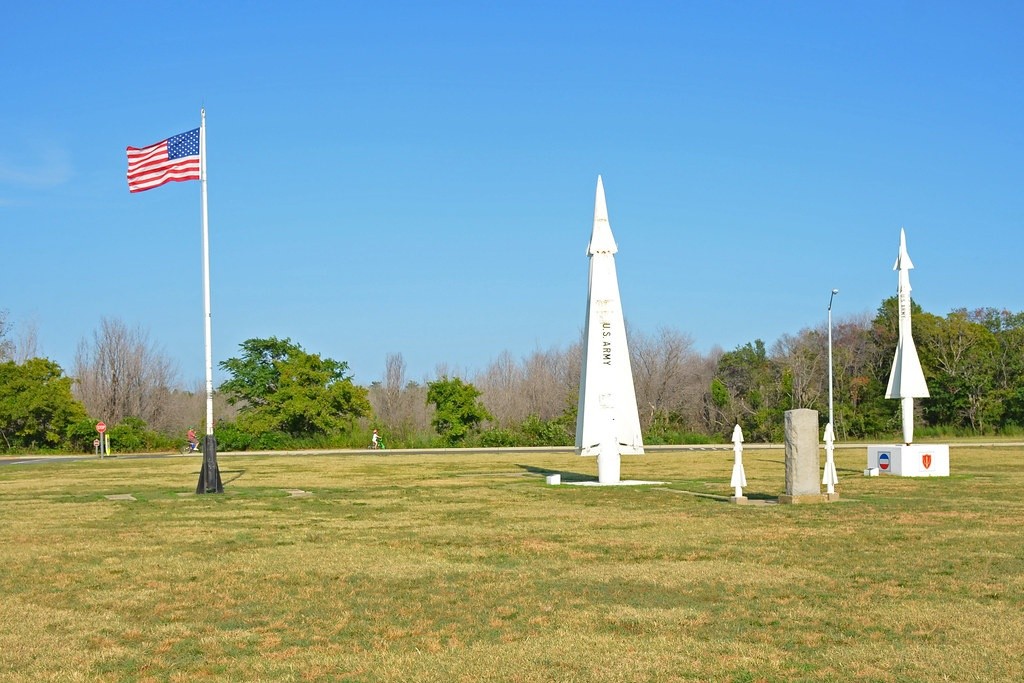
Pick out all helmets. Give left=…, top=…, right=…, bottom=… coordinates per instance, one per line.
left=188, top=425, right=193, bottom=430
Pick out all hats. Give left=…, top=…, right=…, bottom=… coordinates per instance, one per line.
left=373, top=429, right=377, bottom=434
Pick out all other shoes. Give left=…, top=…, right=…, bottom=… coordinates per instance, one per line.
left=193, top=448, right=199, bottom=450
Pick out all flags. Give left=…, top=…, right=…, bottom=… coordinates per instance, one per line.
left=127, top=127, right=204, bottom=194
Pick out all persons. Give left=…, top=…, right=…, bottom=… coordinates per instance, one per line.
left=188, top=426, right=200, bottom=450
left=372, top=430, right=382, bottom=448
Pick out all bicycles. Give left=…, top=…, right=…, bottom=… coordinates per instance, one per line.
left=179, top=441, right=205, bottom=454
left=367, top=437, right=386, bottom=449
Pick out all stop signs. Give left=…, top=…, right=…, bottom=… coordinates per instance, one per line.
left=96, top=422, right=107, bottom=433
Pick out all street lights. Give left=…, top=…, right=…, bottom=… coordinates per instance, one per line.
left=828, top=289, right=838, bottom=443
